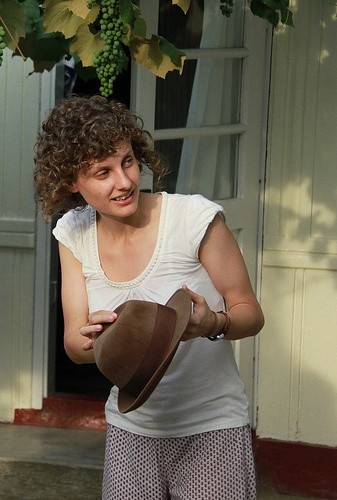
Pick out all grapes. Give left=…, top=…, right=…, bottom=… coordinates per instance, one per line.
left=87, top=0, right=129, bottom=98
left=0, top=26, right=6, bottom=67
left=219, top=0, right=234, bottom=18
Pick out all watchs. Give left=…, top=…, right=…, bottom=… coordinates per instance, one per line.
left=206, top=310, right=231, bottom=341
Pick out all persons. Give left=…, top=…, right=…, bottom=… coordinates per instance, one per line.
left=33, top=96, right=264, bottom=500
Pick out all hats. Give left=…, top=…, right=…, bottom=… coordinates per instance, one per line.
left=90, top=289, right=195, bottom=414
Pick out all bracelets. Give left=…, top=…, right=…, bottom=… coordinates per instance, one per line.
left=201, top=309, right=217, bottom=338
